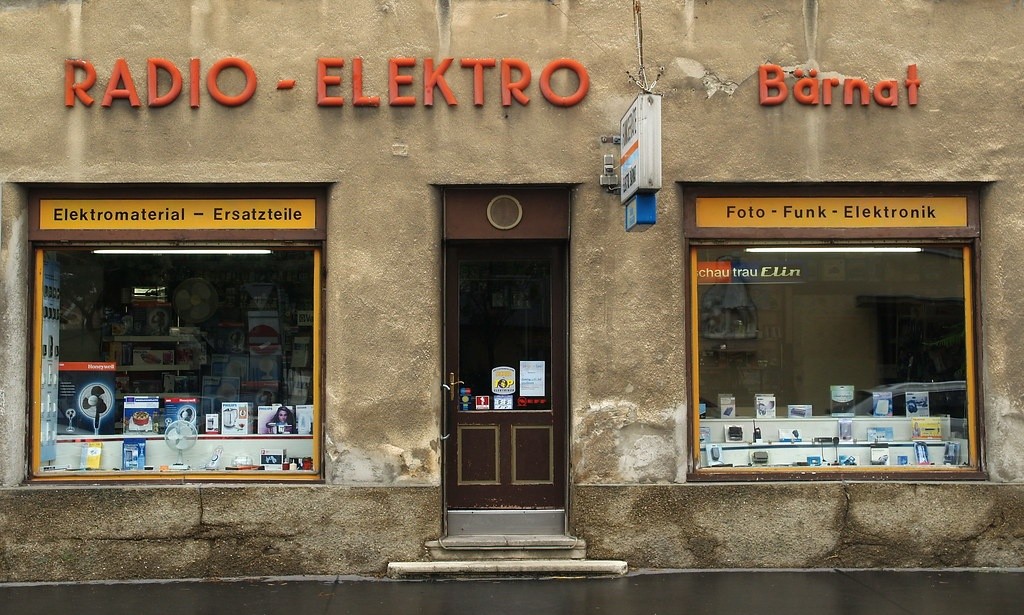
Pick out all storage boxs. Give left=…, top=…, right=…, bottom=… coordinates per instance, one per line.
left=201, top=396, right=221, bottom=418
left=754, top=394, right=775, bottom=418
left=133, top=350, right=174, bottom=365
left=870, top=444, right=890, bottom=466
left=265, top=463, right=289, bottom=471
left=164, top=375, right=198, bottom=393
left=139, top=380, right=163, bottom=393
left=247, top=310, right=279, bottom=344
left=249, top=343, right=282, bottom=356
left=123, top=395, right=159, bottom=434
left=202, top=377, right=240, bottom=402
left=829, top=385, right=855, bottom=417
left=112, top=307, right=171, bottom=335
left=164, top=397, right=200, bottom=428
left=176, top=342, right=207, bottom=365
left=296, top=405, right=314, bottom=435
left=718, top=393, right=735, bottom=418
left=221, top=402, right=253, bottom=434
left=807, top=456, right=821, bottom=467
left=241, top=393, right=276, bottom=413
left=56, top=362, right=116, bottom=435
left=258, top=406, right=292, bottom=435
left=116, top=376, right=129, bottom=394
left=260, top=448, right=286, bottom=466
left=787, top=404, right=812, bottom=418
left=206, top=413, right=220, bottom=434
left=778, top=429, right=802, bottom=442
left=211, top=354, right=250, bottom=381
left=839, top=456, right=858, bottom=467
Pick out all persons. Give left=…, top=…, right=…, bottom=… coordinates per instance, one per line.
left=267, top=407, right=294, bottom=434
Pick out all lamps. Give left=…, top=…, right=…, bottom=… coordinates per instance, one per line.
left=743, top=245, right=924, bottom=254
left=91, top=248, right=273, bottom=254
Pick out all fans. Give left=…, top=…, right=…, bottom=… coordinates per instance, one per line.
left=151, top=311, right=165, bottom=335
left=229, top=332, right=244, bottom=351
left=217, top=384, right=236, bottom=413
left=79, top=383, right=113, bottom=434
left=259, top=359, right=274, bottom=379
left=165, top=420, right=198, bottom=470
left=65, top=409, right=76, bottom=432
left=169, top=277, right=218, bottom=334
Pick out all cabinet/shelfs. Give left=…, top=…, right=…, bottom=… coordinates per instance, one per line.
left=103, top=335, right=201, bottom=428
